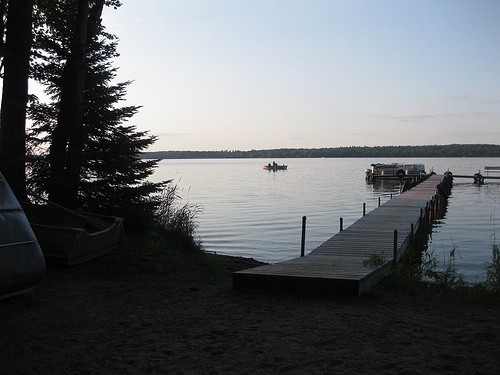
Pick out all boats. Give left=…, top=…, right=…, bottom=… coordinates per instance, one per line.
left=264, top=163, right=288, bottom=170
left=365, top=163, right=427, bottom=181
